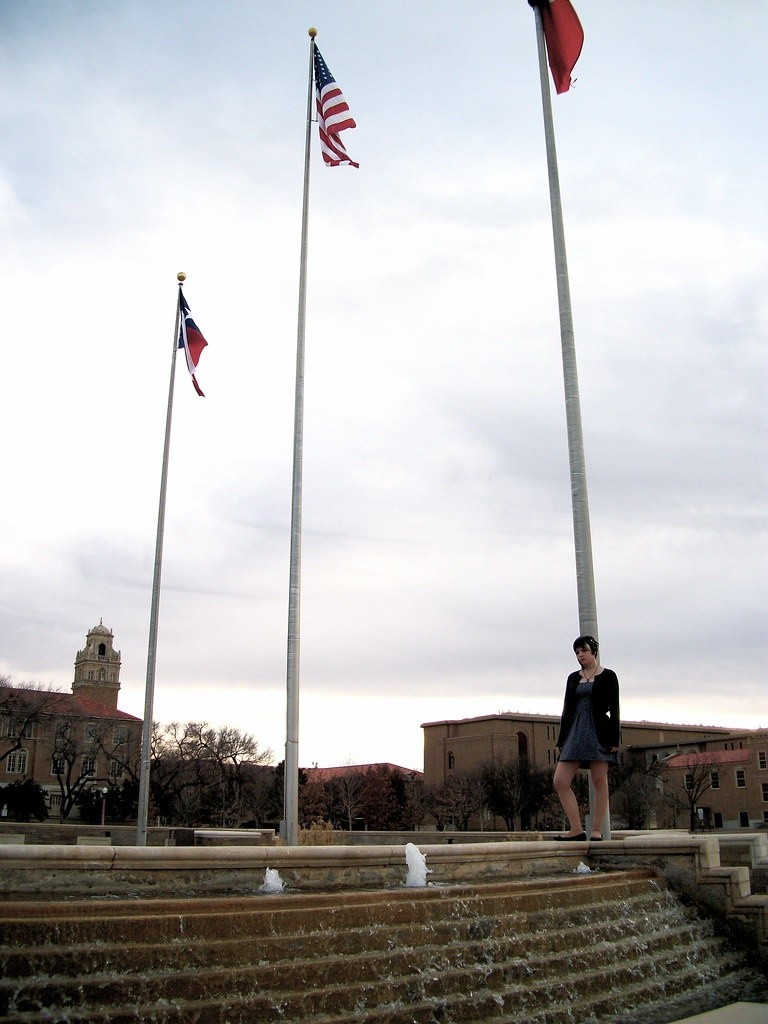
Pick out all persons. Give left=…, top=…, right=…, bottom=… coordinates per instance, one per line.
left=552, top=635, right=621, bottom=841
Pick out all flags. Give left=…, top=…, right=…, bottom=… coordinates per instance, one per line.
left=312, top=40, right=360, bottom=169
left=527, top=0, right=586, bottom=95
left=178, top=282, right=210, bottom=397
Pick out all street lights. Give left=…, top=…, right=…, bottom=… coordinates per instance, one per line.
left=101, top=786, right=107, bottom=824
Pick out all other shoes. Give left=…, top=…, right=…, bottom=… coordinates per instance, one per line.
left=552, top=832, right=587, bottom=841
left=590, top=834, right=604, bottom=840
